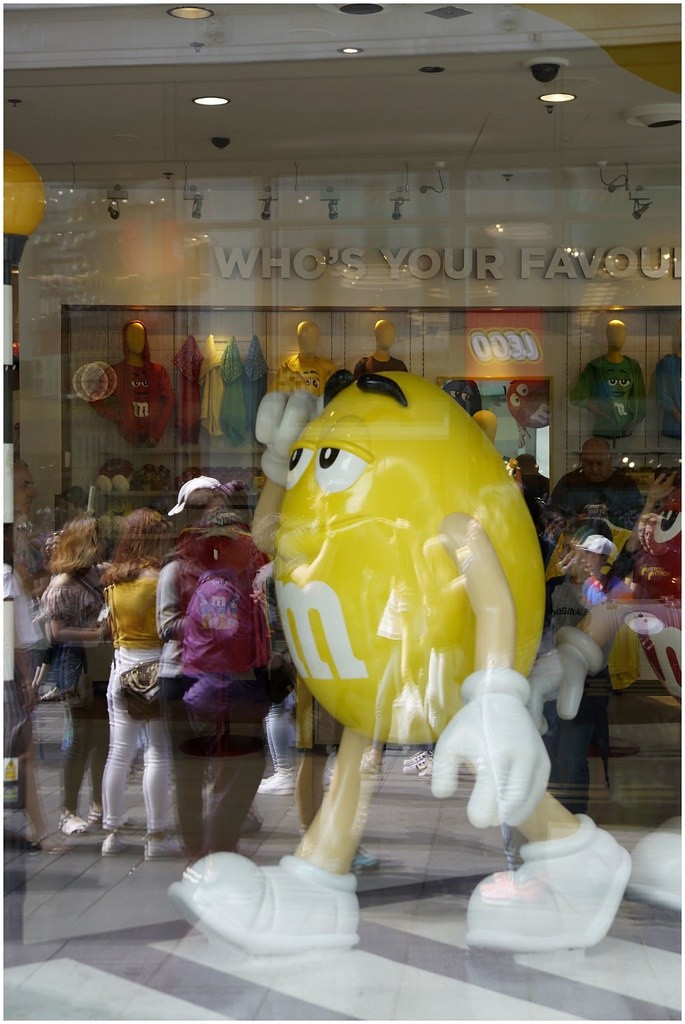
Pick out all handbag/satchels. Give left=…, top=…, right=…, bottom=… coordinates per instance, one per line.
left=66, top=673, right=94, bottom=709
left=119, top=659, right=164, bottom=720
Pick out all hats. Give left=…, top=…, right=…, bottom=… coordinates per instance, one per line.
left=579, top=536, right=618, bottom=557
left=168, top=475, right=221, bottom=516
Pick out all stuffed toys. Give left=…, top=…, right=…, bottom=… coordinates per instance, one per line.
left=179, top=371, right=630, bottom=957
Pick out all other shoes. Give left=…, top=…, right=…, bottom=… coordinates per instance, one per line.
left=41, top=681, right=75, bottom=701
left=202, top=808, right=262, bottom=835
left=58, top=811, right=89, bottom=835
left=145, top=835, right=184, bottom=861
left=88, top=809, right=103, bottom=824
left=101, top=830, right=127, bottom=854
left=349, top=845, right=380, bottom=869
left=256, top=767, right=296, bottom=796
left=30, top=829, right=70, bottom=854
left=358, top=751, right=382, bottom=774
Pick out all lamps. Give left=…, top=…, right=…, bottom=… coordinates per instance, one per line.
left=320, top=191, right=340, bottom=219
left=183, top=185, right=204, bottom=219
left=107, top=191, right=128, bottom=220
left=258, top=192, right=279, bottom=220
left=629, top=192, right=654, bottom=221
left=389, top=164, right=411, bottom=220
left=600, top=163, right=630, bottom=193
left=420, top=168, right=444, bottom=194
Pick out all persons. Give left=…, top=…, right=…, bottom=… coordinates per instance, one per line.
left=551, top=436, right=675, bottom=552
left=352, top=319, right=408, bottom=382
left=502, top=515, right=681, bottom=876
left=88, top=320, right=173, bottom=447
left=8, top=458, right=456, bottom=872
left=266, top=321, right=337, bottom=396
left=653, top=320, right=682, bottom=440
left=513, top=455, right=549, bottom=530
left=570, top=320, right=646, bottom=439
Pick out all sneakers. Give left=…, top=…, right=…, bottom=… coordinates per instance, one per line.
left=403, top=750, right=434, bottom=778
